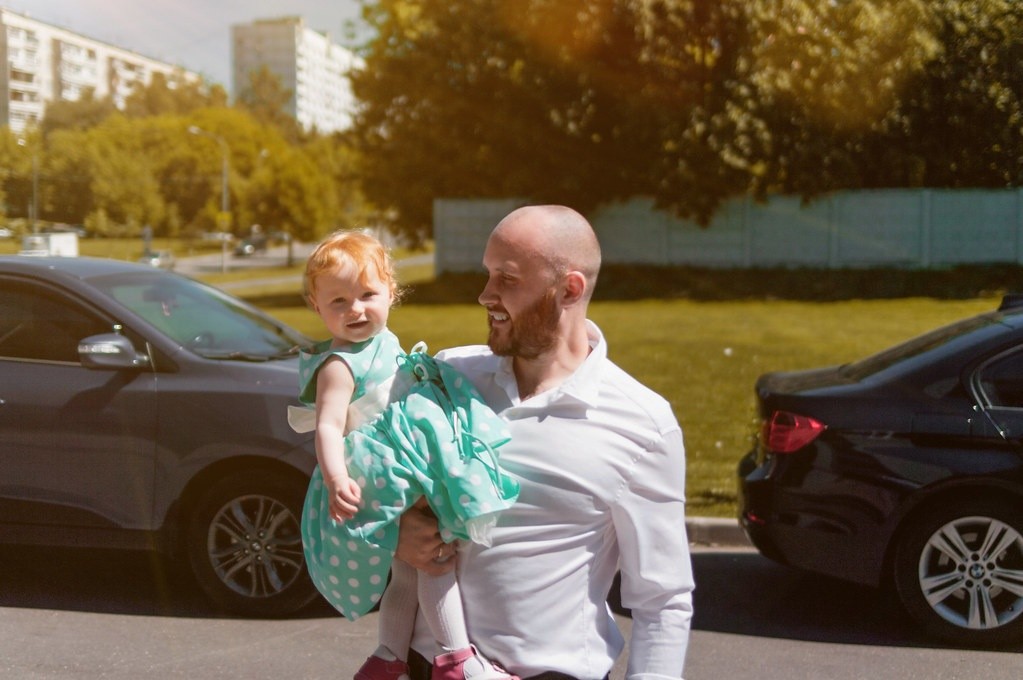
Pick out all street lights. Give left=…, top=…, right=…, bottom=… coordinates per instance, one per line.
left=189, top=125, right=230, bottom=275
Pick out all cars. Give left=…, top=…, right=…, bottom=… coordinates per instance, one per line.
left=736, top=295, right=1023, bottom=650
left=235, top=232, right=289, bottom=255
left=0, top=256, right=339, bottom=618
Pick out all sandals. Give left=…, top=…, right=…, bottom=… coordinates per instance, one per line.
left=353, top=644, right=411, bottom=680
left=431, top=641, right=521, bottom=680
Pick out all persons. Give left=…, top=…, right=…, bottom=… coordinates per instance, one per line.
left=299, top=228, right=521, bottom=680
left=394, top=204, right=697, bottom=679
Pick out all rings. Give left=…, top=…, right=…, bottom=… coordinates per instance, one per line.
left=439, top=548, right=443, bottom=556
